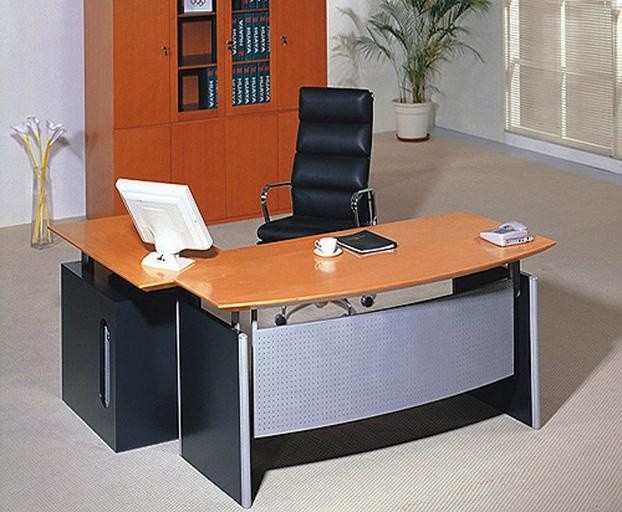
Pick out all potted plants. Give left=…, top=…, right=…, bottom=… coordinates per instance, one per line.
left=337, top=0, right=498, bottom=144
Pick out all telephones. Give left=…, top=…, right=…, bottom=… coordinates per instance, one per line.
left=480, top=221, right=534, bottom=247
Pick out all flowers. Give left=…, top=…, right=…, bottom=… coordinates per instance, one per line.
left=9, top=115, right=68, bottom=250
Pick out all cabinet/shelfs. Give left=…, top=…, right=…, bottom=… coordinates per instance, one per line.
left=79, top=0, right=329, bottom=236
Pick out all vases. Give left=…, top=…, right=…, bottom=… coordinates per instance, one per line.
left=29, top=164, right=55, bottom=250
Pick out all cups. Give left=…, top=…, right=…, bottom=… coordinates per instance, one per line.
left=314, top=237, right=337, bottom=254
left=315, top=262, right=335, bottom=273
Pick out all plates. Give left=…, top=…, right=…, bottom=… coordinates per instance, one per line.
left=314, top=256, right=339, bottom=263
left=312, top=248, right=343, bottom=258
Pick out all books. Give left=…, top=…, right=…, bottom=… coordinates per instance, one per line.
left=336, top=244, right=396, bottom=258
left=198, top=0, right=271, bottom=109
left=334, top=230, right=398, bottom=254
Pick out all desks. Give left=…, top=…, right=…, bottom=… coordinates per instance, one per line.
left=44, top=211, right=557, bottom=511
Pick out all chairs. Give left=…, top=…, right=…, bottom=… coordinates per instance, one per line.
left=250, top=81, right=384, bottom=327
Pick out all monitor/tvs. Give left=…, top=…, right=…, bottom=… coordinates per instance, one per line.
left=113, top=176, right=214, bottom=273
